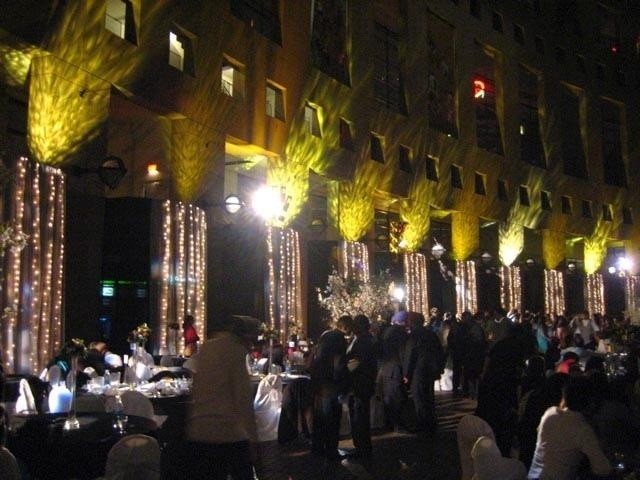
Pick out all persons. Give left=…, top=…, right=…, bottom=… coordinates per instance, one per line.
left=45, top=306, right=639, bottom=480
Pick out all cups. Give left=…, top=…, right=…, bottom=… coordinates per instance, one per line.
left=110, top=372, right=121, bottom=385
left=127, top=377, right=190, bottom=398
left=87, top=376, right=105, bottom=391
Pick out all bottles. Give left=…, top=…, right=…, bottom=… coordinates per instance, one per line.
left=104, top=369, right=110, bottom=385
left=48, top=385, right=72, bottom=412
